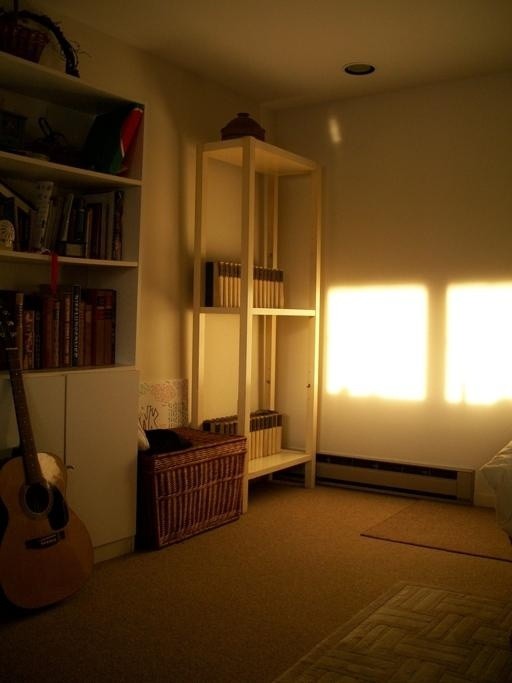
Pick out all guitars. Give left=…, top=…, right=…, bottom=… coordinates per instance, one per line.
left=0, top=305, right=94, bottom=610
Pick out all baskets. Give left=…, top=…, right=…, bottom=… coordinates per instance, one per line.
left=0, top=19, right=51, bottom=64
left=138, top=420, right=245, bottom=549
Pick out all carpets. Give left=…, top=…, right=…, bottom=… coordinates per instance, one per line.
left=360, top=499, right=512, bottom=561
left=270, top=579, right=511, bottom=681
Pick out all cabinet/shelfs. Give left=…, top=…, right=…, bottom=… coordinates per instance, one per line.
left=0, top=48, right=148, bottom=567
left=191, top=135, right=323, bottom=515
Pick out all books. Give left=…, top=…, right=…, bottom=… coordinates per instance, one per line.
left=0, top=97, right=143, bottom=371
